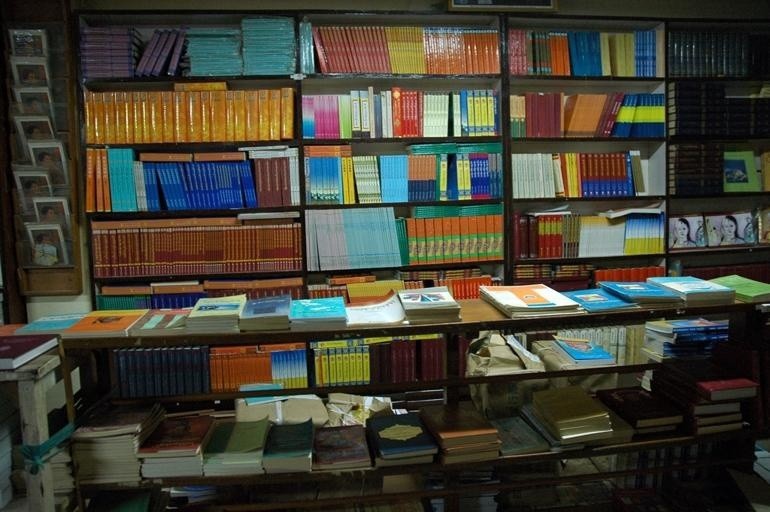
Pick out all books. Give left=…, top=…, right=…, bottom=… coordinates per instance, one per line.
left=80, top=19, right=301, bottom=308
left=507, top=26, right=666, bottom=292
left=303, top=19, right=505, bottom=302
left=2, top=275, right=770, bottom=511
left=666, top=25, right=769, bottom=282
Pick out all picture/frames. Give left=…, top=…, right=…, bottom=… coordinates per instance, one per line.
left=446, top=0, right=560, bottom=14
